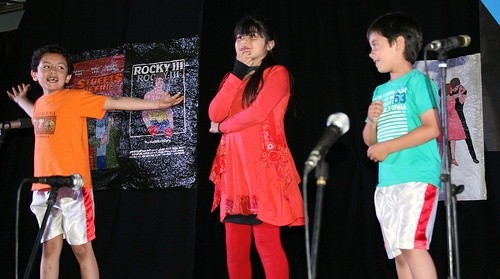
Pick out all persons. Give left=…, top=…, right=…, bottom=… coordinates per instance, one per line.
left=3, top=45, right=184, bottom=279
left=360, top=11, right=443, bottom=279
left=206, top=15, right=310, bottom=279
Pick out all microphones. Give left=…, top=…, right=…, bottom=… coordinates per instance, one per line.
left=424, top=33, right=470, bottom=55
left=302, top=111, right=350, bottom=178
left=0, top=117, right=32, bottom=129
left=27, top=174, right=84, bottom=192
